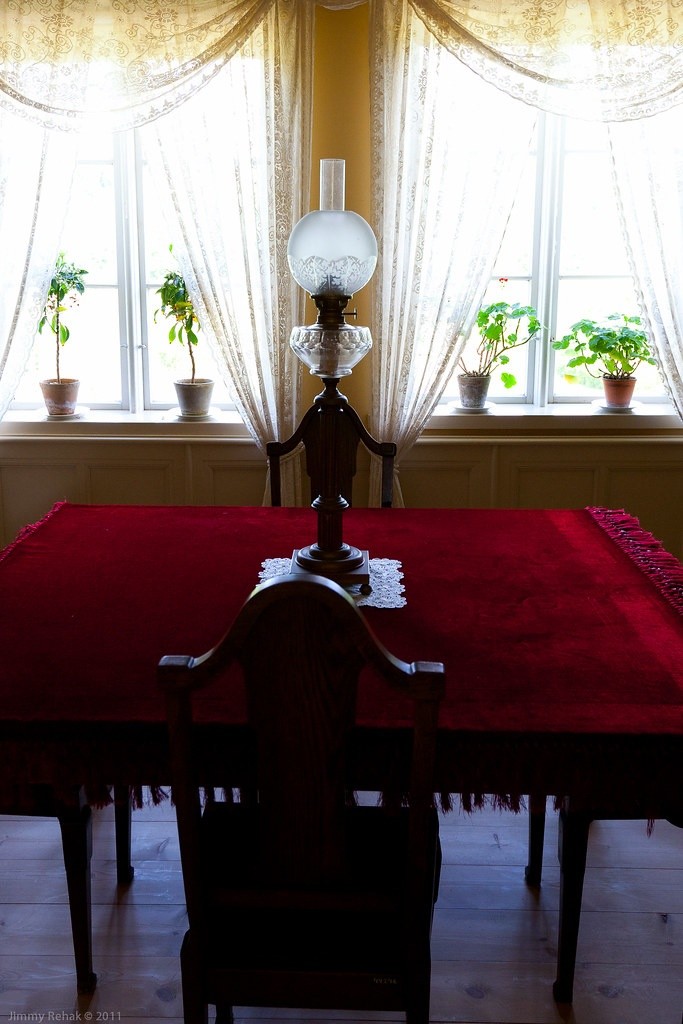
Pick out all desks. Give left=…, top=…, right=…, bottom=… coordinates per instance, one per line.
left=0, top=497, right=683, bottom=1024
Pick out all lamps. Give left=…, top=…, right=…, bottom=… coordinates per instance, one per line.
left=284, top=158, right=382, bottom=597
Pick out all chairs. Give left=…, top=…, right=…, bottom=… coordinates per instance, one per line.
left=263, top=395, right=399, bottom=510
left=152, top=574, right=454, bottom=1024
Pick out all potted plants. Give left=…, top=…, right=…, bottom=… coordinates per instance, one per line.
left=442, top=293, right=550, bottom=413
left=551, top=311, right=659, bottom=412
left=35, top=250, right=92, bottom=420
left=153, top=242, right=222, bottom=422
